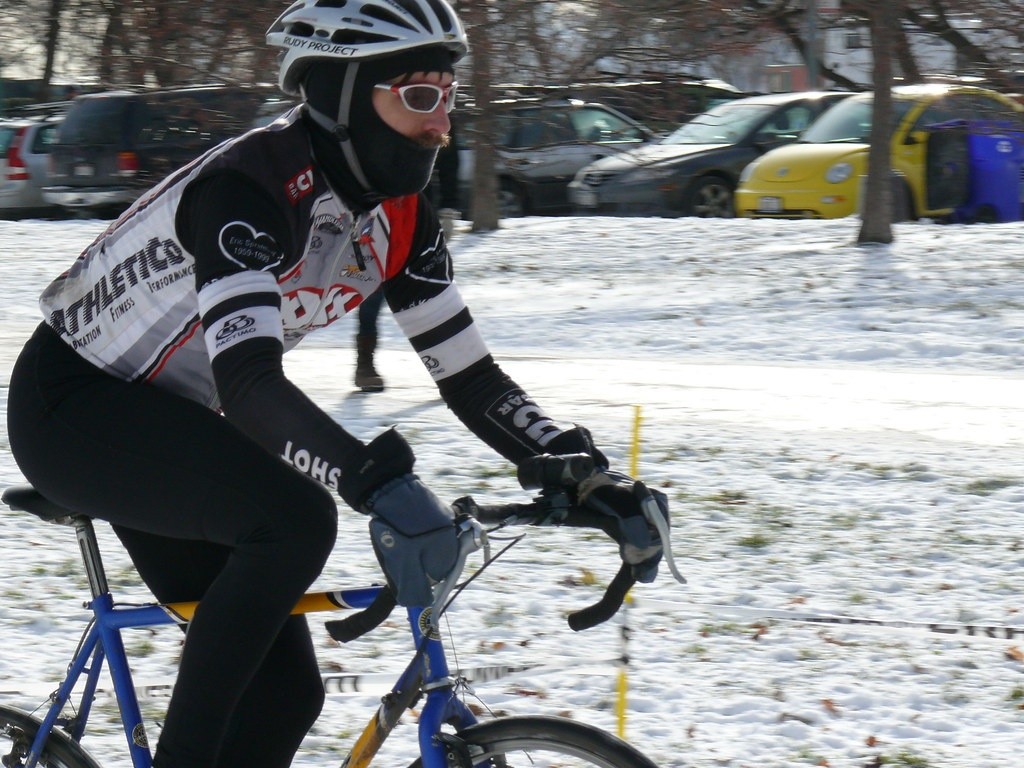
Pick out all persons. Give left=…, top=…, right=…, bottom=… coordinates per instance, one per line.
left=7, top=0, right=669, bottom=768
left=355, top=113, right=458, bottom=391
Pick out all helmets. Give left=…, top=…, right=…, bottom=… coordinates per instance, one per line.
left=264, top=0, right=467, bottom=98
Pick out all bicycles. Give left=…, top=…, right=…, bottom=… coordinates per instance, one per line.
left=0, top=453, right=687, bottom=768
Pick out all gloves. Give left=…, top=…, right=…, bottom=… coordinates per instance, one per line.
left=519, top=444, right=670, bottom=584
left=338, top=426, right=457, bottom=609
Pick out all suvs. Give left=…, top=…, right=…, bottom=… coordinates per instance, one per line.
left=446, top=72, right=754, bottom=219
left=1, top=83, right=300, bottom=219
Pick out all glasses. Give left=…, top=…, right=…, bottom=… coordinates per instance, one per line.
left=377, top=81, right=459, bottom=113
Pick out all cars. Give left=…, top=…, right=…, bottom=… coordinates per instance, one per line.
left=565, top=88, right=957, bottom=218
left=735, top=75, right=1024, bottom=223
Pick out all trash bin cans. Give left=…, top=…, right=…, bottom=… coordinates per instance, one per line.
left=924, top=117, right=1023, bottom=222
